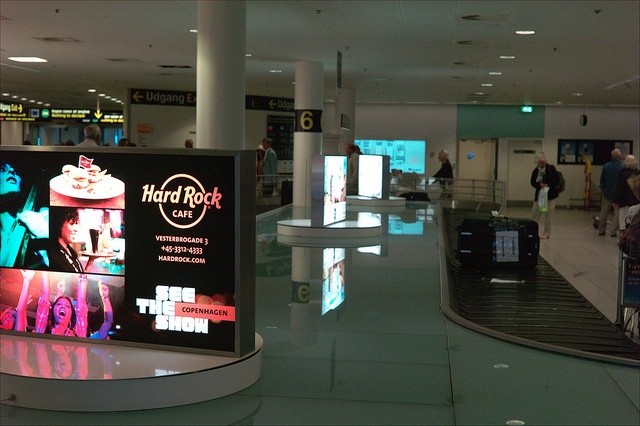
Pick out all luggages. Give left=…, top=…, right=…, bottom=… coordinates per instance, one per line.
left=453, top=216, right=540, bottom=269
left=399, top=192, right=431, bottom=201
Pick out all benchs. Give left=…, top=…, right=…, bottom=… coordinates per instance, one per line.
left=566, top=196, right=601, bottom=210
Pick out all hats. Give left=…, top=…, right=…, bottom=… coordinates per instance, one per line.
left=256, top=144, right=265, bottom=152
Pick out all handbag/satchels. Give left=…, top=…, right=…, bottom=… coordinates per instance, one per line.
left=618, top=209, right=640, bottom=257
left=538, top=186, right=550, bottom=212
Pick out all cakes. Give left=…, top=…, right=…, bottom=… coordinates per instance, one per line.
left=49, top=154, right=125, bottom=210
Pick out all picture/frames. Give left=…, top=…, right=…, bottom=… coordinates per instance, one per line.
left=557, top=138, right=633, bottom=165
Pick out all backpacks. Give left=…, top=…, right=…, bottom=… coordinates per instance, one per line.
left=546, top=165, right=565, bottom=191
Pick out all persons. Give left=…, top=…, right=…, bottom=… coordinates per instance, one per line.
left=426, top=149, right=453, bottom=201
left=0, top=163, right=49, bottom=271
left=256, top=144, right=265, bottom=182
left=0, top=269, right=35, bottom=332
left=598, top=148, right=624, bottom=237
left=346, top=144, right=358, bottom=195
left=261, top=138, right=278, bottom=195
left=355, top=145, right=363, bottom=154
left=0, top=337, right=113, bottom=381
left=530, top=154, right=561, bottom=240
left=185, top=139, right=193, bottom=148
left=119, top=139, right=128, bottom=146
left=49, top=206, right=102, bottom=273
left=610, top=155, right=640, bottom=235
left=53, top=279, right=113, bottom=339
left=76, top=124, right=101, bottom=146
left=35, top=271, right=88, bottom=338
